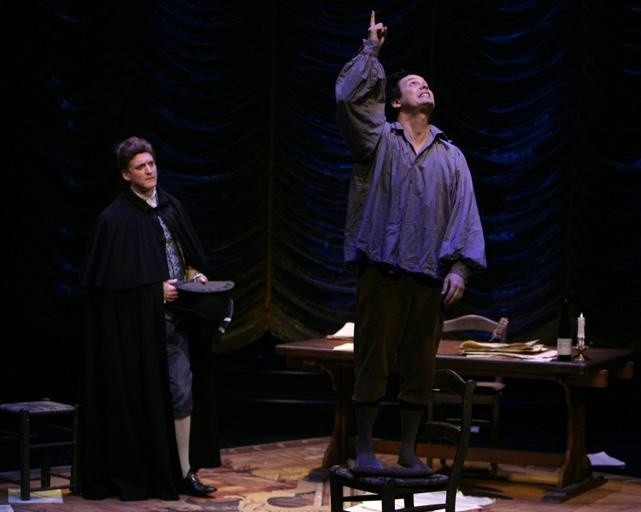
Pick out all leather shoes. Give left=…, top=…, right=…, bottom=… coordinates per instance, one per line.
left=177, top=469, right=217, bottom=495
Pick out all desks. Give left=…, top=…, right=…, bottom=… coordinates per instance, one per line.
left=277, top=332, right=629, bottom=504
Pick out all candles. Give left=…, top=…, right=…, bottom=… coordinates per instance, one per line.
left=577, top=317, right=587, bottom=338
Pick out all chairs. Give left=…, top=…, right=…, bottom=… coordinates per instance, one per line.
left=327, top=312, right=512, bottom=511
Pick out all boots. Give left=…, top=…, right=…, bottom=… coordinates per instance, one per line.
left=354, top=404, right=426, bottom=471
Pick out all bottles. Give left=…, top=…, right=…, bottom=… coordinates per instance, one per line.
left=556, top=296, right=573, bottom=360
left=477, top=317, right=509, bottom=382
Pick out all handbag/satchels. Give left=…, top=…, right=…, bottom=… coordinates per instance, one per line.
left=345, top=162, right=371, bottom=240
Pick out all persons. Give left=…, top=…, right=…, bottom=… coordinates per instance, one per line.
left=328, top=10, right=492, bottom=477
left=82, top=136, right=219, bottom=499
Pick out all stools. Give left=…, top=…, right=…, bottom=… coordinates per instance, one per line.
left=1, top=395, right=78, bottom=501
